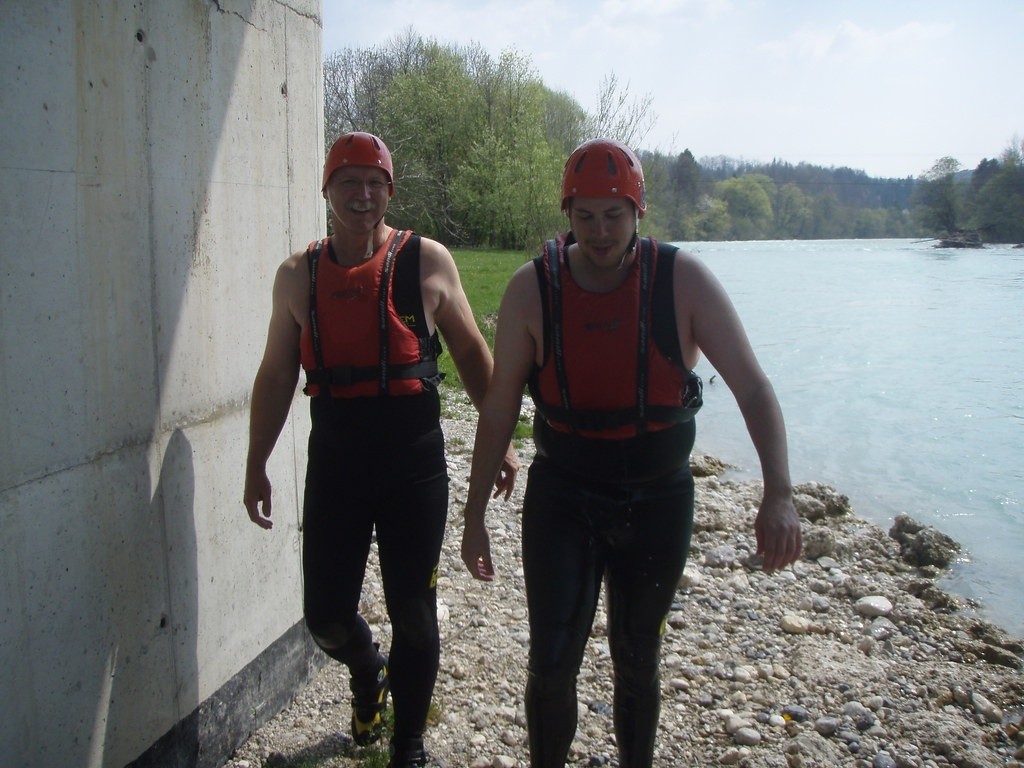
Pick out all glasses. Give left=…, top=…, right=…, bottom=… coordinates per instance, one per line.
left=332, top=176, right=392, bottom=192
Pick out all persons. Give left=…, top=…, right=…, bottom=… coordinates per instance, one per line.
left=460, top=137, right=801, bottom=768
left=243, top=132, right=519, bottom=768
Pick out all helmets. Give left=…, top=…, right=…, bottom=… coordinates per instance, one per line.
left=560, top=138, right=647, bottom=220
left=321, top=131, right=394, bottom=199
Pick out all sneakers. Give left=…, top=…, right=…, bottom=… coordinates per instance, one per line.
left=385, top=730, right=423, bottom=768
left=349, top=656, right=389, bottom=746
